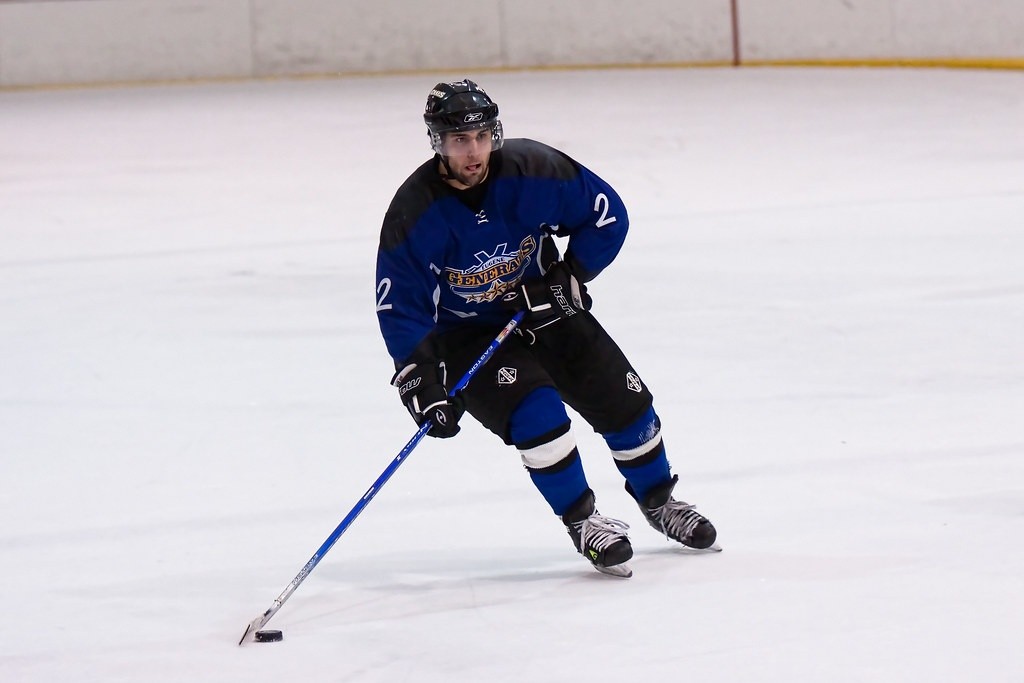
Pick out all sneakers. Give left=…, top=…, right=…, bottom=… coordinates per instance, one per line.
left=556, top=488, right=633, bottom=578
left=625, top=475, right=723, bottom=553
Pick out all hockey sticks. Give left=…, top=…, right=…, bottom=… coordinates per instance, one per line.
left=237, top=304, right=527, bottom=646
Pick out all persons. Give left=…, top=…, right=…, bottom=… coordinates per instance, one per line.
left=376, top=79, right=723, bottom=577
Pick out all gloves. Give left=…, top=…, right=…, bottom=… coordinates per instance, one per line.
left=502, top=261, right=592, bottom=348
left=390, top=360, right=465, bottom=439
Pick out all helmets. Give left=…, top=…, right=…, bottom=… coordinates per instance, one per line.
left=424, top=79, right=504, bottom=158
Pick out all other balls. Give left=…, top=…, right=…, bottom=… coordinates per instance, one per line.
left=255, top=630, right=283, bottom=642
